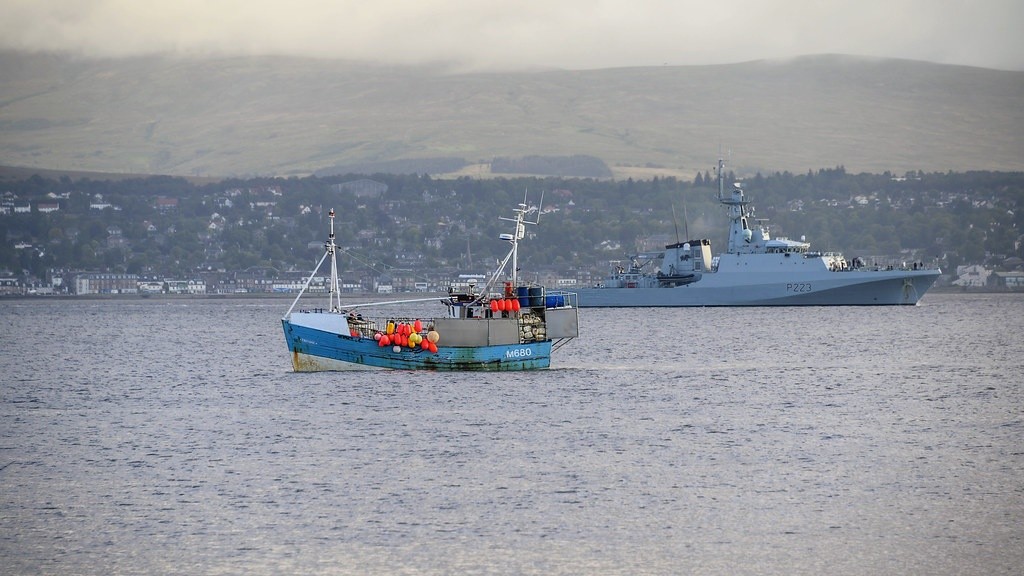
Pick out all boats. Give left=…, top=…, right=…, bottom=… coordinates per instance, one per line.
left=280, top=187, right=578, bottom=373
left=542, top=149, right=942, bottom=307
